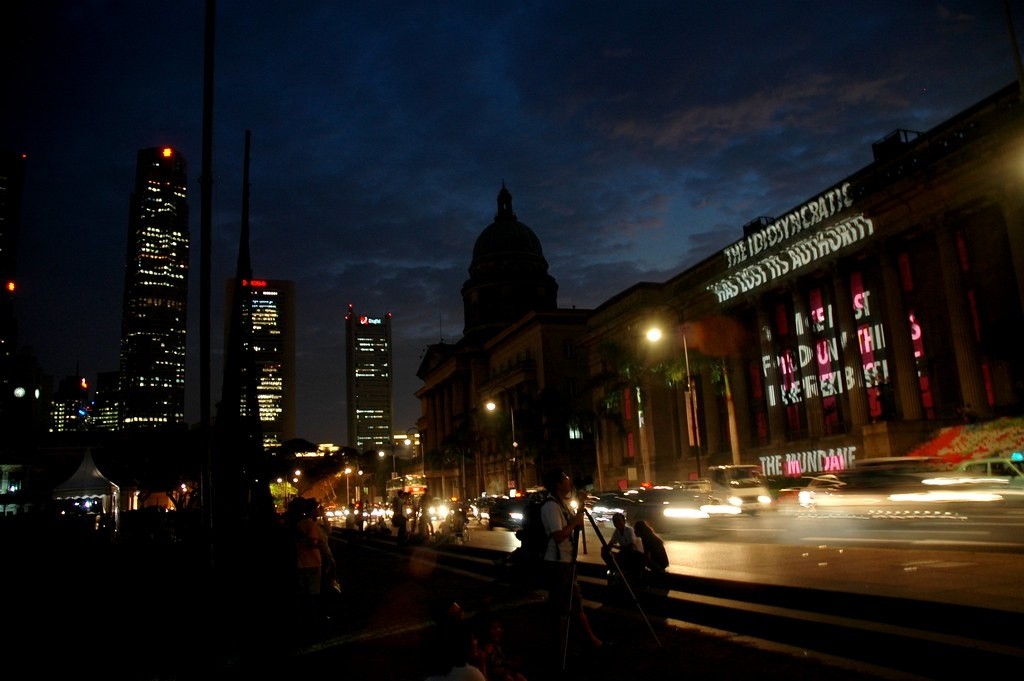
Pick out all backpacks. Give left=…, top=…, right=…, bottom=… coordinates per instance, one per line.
left=521, top=497, right=559, bottom=562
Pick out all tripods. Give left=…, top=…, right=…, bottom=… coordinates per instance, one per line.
left=560, top=504, right=663, bottom=672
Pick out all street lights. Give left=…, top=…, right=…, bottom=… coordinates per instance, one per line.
left=646, top=304, right=704, bottom=479
left=486, top=385, right=522, bottom=490
left=403, top=426, right=426, bottom=483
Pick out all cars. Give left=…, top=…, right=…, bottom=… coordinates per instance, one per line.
left=471, top=465, right=774, bottom=534
left=954, top=453, right=1024, bottom=492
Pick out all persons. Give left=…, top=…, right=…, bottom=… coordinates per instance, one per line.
left=392, top=465, right=668, bottom=681
left=279, top=495, right=342, bottom=594
